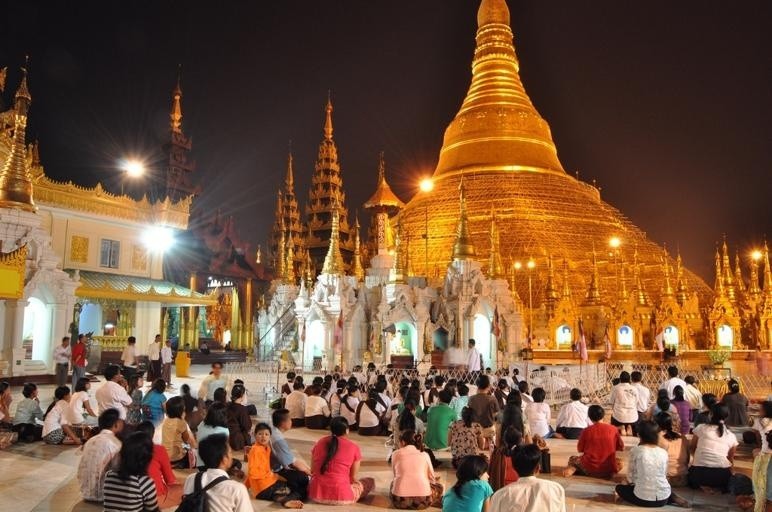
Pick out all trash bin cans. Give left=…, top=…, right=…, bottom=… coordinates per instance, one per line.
left=175, top=351, right=191, bottom=377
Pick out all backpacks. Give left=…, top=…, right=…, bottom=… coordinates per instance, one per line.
left=174, top=470, right=229, bottom=512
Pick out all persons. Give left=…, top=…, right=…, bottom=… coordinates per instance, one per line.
left=71, top=334, right=87, bottom=395
left=161, top=340, right=177, bottom=385
left=0, top=360, right=772, bottom=511
left=147, top=335, right=161, bottom=382
left=53, top=337, right=72, bottom=387
left=465, top=340, right=482, bottom=385
left=199, top=341, right=209, bottom=356
left=120, top=336, right=140, bottom=395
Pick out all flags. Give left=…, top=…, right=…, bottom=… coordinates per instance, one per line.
left=576, top=315, right=592, bottom=360
left=489, top=305, right=499, bottom=337
left=603, top=327, right=612, bottom=361
left=333, top=310, right=345, bottom=346
left=655, top=326, right=664, bottom=352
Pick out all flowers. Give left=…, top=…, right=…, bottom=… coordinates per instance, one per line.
left=703, top=346, right=733, bottom=363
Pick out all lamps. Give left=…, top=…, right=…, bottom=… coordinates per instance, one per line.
left=520, top=347, right=534, bottom=361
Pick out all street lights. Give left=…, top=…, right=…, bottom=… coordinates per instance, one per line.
left=512, top=260, right=536, bottom=349
left=750, top=250, right=764, bottom=287
left=608, top=235, right=621, bottom=288
left=419, top=177, right=438, bottom=279
left=118, top=159, right=144, bottom=195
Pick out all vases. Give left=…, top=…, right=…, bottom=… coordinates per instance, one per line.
left=713, top=362, right=725, bottom=375
